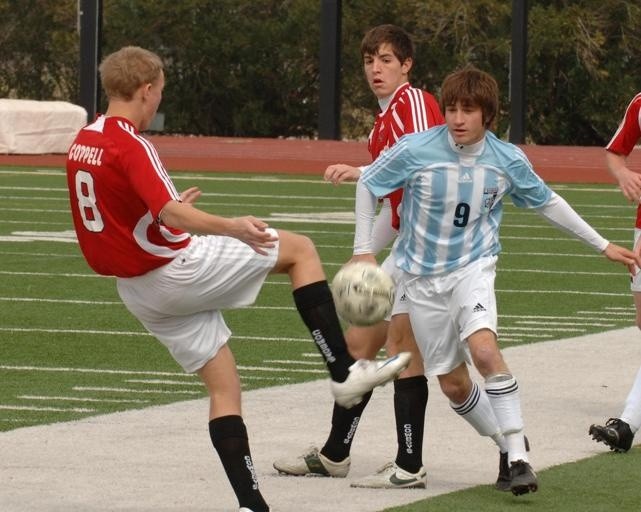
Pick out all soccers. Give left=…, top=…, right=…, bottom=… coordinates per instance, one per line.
left=330, top=260, right=396, bottom=326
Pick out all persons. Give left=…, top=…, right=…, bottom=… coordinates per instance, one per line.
left=65, top=44, right=413, bottom=512
left=273, top=25, right=447, bottom=490
left=340, top=63, right=640, bottom=496
left=587, top=92, right=640, bottom=452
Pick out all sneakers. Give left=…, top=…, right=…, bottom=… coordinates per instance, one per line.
left=273, top=448, right=352, bottom=479
left=495, top=436, right=537, bottom=496
left=330, top=351, right=412, bottom=410
left=590, top=416, right=635, bottom=453
left=350, top=459, right=428, bottom=490
